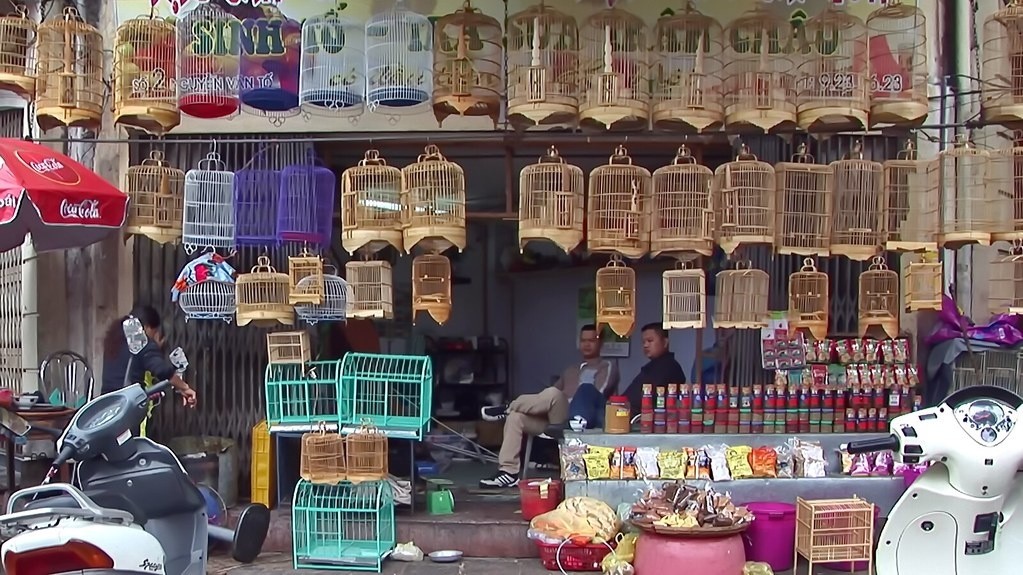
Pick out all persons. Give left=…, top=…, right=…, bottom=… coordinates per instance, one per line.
left=101, top=304, right=197, bottom=442
left=328, top=319, right=383, bottom=395
left=480, top=325, right=620, bottom=488
left=544, top=322, right=686, bottom=440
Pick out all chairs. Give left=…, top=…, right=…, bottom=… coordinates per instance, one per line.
left=522, top=357, right=612, bottom=480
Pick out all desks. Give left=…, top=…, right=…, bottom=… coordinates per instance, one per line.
left=0, top=406, right=76, bottom=496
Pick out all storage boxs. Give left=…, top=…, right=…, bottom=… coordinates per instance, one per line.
left=535, top=538, right=618, bottom=571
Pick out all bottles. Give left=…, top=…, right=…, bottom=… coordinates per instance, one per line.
left=604, top=396, right=630, bottom=434
left=640, top=384, right=913, bottom=435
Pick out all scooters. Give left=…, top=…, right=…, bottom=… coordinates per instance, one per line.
left=847, top=383, right=1023, bottom=575
left=0, top=318, right=208, bottom=575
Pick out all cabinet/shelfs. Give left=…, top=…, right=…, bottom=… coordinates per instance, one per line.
left=424, top=335, right=510, bottom=429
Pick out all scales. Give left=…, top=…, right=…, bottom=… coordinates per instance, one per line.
left=426, top=479, right=455, bottom=515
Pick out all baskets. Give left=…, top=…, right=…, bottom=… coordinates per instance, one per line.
left=536, top=538, right=616, bottom=571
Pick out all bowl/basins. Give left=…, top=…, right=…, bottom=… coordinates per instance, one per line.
left=12, top=396, right=39, bottom=410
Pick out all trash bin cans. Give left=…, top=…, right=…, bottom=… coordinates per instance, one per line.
left=166, top=431, right=239, bottom=512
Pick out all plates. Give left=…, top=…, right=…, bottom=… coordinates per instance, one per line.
left=428, top=549, right=463, bottom=562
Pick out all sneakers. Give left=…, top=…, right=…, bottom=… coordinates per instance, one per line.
left=481, top=404, right=510, bottom=421
left=479, top=470, right=520, bottom=489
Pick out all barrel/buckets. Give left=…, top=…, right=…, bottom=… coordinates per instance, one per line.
left=518, top=478, right=562, bottom=521
left=738, top=502, right=797, bottom=571
left=821, top=505, right=880, bottom=571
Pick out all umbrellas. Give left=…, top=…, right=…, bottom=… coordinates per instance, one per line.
left=0, top=135, right=130, bottom=254
